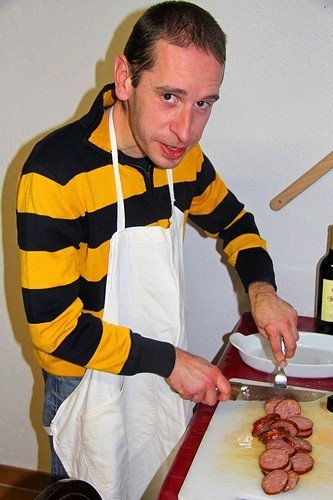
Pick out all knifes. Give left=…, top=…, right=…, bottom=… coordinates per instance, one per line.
left=171, top=381, right=327, bottom=403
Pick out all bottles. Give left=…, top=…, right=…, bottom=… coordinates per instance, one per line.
left=315, top=224, right=333, bottom=335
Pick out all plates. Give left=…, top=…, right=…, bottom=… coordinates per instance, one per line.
left=229, top=331, right=333, bottom=378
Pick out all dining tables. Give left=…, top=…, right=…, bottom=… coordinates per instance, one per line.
left=155, top=312, right=333, bottom=500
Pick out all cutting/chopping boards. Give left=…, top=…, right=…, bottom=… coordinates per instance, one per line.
left=178, top=378, right=333, bottom=500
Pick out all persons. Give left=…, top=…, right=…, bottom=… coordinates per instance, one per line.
left=13, top=1, right=299, bottom=500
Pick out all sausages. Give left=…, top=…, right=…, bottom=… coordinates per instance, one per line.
left=252, top=398, right=314, bottom=494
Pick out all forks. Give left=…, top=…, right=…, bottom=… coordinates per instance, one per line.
left=274, top=336, right=287, bottom=388
